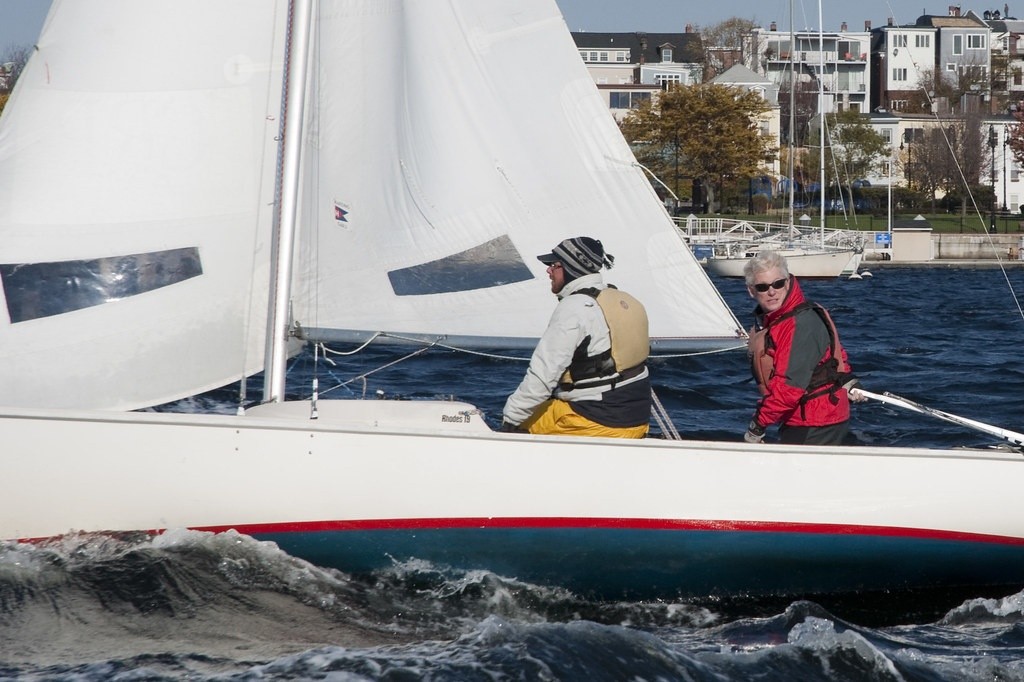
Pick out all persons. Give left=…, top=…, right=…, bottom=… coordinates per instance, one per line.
left=1008, top=248, right=1014, bottom=260
left=496, top=236, right=653, bottom=438
left=742, top=251, right=868, bottom=445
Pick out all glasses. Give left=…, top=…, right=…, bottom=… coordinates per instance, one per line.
left=550, top=263, right=564, bottom=270
left=751, top=278, right=788, bottom=292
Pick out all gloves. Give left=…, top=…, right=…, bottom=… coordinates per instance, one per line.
left=485, top=412, right=518, bottom=431
left=743, top=420, right=766, bottom=443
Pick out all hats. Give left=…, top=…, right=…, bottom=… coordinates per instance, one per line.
left=552, top=237, right=614, bottom=280
left=537, top=253, right=558, bottom=266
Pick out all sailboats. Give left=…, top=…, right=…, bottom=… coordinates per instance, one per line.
left=693, top=0, right=870, bottom=280
left=0, top=0, right=1024, bottom=624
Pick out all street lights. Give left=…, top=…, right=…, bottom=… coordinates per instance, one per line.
left=900, top=132, right=911, bottom=188
left=1000, top=123, right=1010, bottom=216
left=985, top=123, right=998, bottom=233
